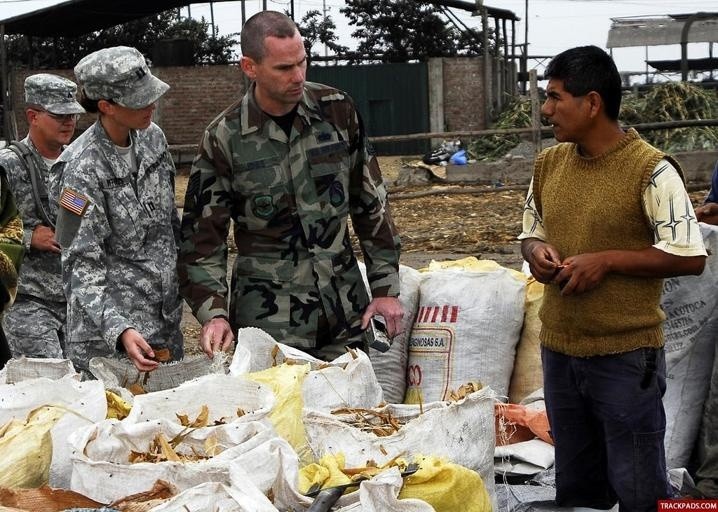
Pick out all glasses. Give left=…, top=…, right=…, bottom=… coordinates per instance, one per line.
left=25, top=107, right=80, bottom=121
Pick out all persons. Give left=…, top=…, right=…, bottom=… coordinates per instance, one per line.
left=518, top=45, right=708, bottom=512
left=0, top=73, right=87, bottom=359
left=0, top=165, right=22, bottom=365
left=695, top=160, right=718, bottom=225
left=48, top=46, right=182, bottom=381
left=177, top=10, right=404, bottom=387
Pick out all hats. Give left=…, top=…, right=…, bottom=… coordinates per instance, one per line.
left=74, top=46, right=171, bottom=109
left=24, top=73, right=87, bottom=115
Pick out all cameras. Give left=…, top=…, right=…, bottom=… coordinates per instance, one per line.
left=363, top=318, right=390, bottom=352
